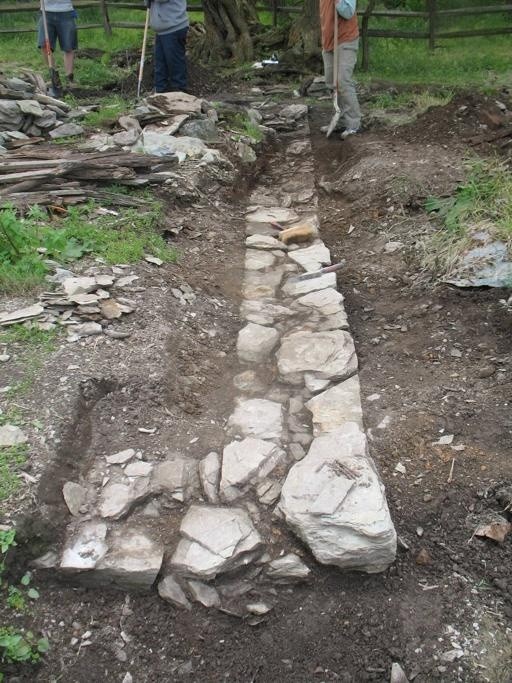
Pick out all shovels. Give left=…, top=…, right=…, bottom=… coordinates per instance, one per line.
left=41, top=0, right=63, bottom=97
left=326, top=7, right=342, bottom=138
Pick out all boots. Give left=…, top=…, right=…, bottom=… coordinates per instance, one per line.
left=319, top=112, right=360, bottom=139
left=66, top=73, right=80, bottom=92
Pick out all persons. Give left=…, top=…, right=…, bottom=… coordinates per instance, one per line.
left=35, top=0, right=80, bottom=85
left=140, top=0, right=192, bottom=96
left=318, top=0, right=363, bottom=140
